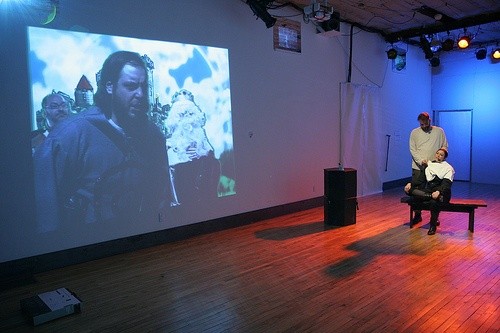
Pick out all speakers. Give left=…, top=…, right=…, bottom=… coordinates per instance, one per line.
left=324, top=167, right=358, bottom=226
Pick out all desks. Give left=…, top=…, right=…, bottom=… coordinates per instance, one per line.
left=323, top=168, right=357, bottom=226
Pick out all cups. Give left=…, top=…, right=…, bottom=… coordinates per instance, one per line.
left=339, top=161, right=345, bottom=170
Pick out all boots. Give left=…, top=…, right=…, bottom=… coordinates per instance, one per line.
left=425, top=192, right=444, bottom=204
left=428, top=217, right=438, bottom=235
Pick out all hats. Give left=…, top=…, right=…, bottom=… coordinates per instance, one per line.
left=418, top=112, right=429, bottom=119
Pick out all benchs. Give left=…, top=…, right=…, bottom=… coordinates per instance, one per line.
left=408, top=199, right=487, bottom=233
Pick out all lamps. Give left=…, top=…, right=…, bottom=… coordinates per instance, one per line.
left=248, top=0, right=276, bottom=28
left=458, top=28, right=471, bottom=49
left=475, top=44, right=487, bottom=60
left=423, top=38, right=454, bottom=67
left=385, top=43, right=397, bottom=60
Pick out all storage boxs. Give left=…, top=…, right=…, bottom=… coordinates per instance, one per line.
left=19, top=288, right=81, bottom=328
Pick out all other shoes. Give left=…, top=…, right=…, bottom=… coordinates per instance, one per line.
left=431, top=219, right=440, bottom=226
left=412, top=211, right=422, bottom=225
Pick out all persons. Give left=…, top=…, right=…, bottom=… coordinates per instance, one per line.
left=405, top=112, right=454, bottom=234
left=36, top=51, right=219, bottom=233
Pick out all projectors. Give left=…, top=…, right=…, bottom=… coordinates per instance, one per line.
left=303, top=3, right=334, bottom=22
